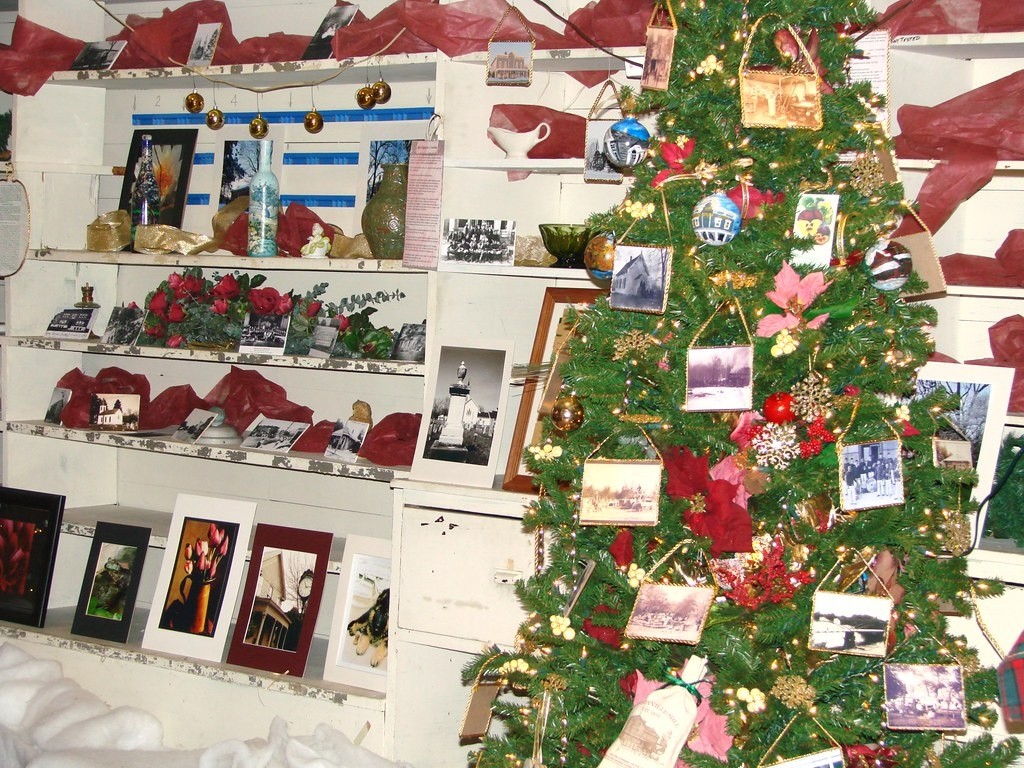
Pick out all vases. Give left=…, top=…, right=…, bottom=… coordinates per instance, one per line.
left=247, top=141, right=279, bottom=257
left=362, top=164, right=409, bottom=259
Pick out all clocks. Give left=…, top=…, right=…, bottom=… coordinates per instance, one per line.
left=299, top=576, right=313, bottom=598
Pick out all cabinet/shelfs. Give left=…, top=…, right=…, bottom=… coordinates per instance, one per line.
left=386, top=32, right=1024, bottom=768
left=0, top=52, right=437, bottom=712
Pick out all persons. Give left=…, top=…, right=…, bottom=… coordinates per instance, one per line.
left=447, top=223, right=502, bottom=263
left=844, top=451, right=899, bottom=505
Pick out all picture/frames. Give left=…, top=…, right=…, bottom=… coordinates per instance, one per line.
left=503, top=286, right=611, bottom=494
left=1, top=486, right=66, bottom=628
left=323, top=533, right=392, bottom=693
left=119, top=128, right=198, bottom=251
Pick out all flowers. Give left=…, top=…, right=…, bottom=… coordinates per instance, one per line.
left=122, top=266, right=406, bottom=360
left=581, top=24, right=905, bottom=768
